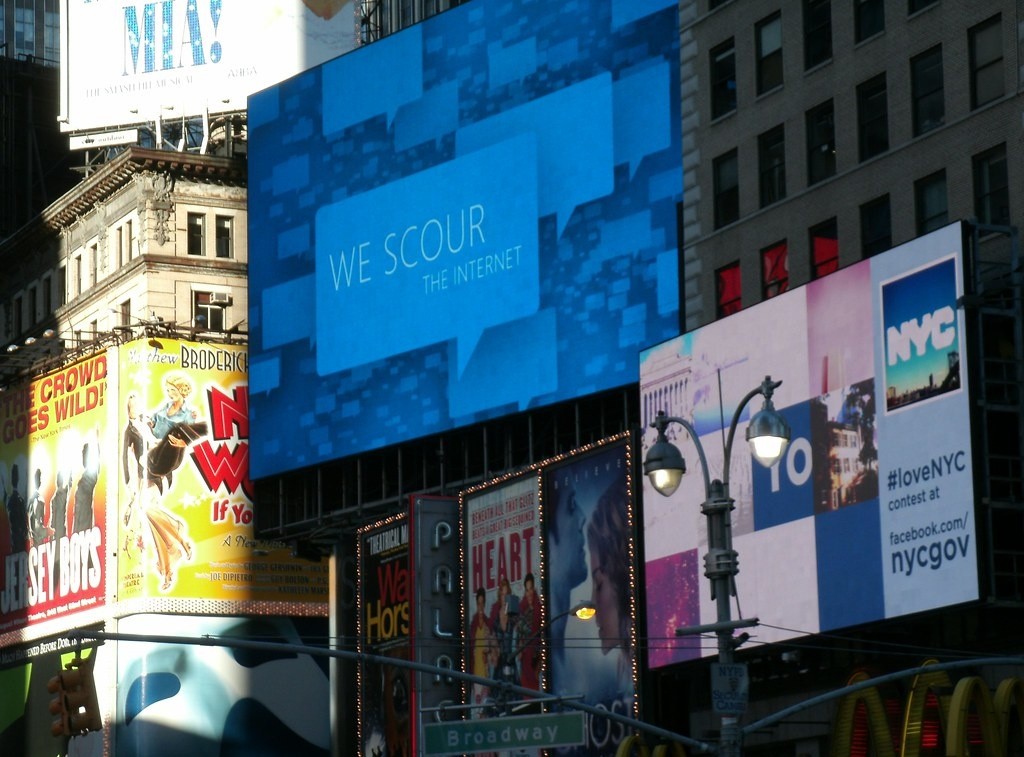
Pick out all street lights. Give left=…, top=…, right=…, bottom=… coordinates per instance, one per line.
left=643, top=374, right=792, bottom=757
left=493, top=594, right=597, bottom=715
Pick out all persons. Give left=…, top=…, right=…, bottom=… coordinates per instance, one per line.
left=468, top=573, right=545, bottom=717
left=544, top=467, right=636, bottom=711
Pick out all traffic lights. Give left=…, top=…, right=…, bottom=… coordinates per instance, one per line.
left=58, top=658, right=103, bottom=735
